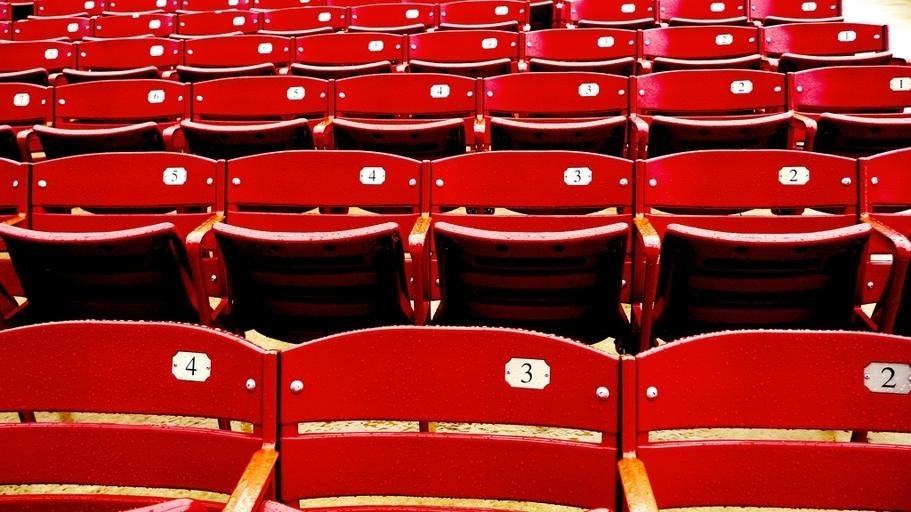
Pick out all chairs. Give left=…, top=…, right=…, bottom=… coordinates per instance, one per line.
left=249, top=326, right=637, bottom=512
left=0, top=321, right=280, bottom=512
left=622, top=330, right=909, bottom=509
left=1, top=0, right=910, bottom=339
